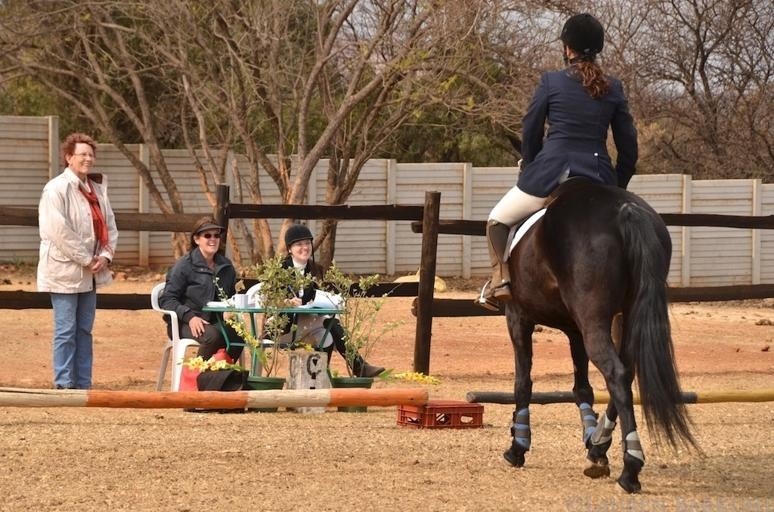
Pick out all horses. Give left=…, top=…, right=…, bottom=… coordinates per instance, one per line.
left=501, top=175, right=702, bottom=493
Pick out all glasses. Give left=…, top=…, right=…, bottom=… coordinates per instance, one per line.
left=289, top=241, right=314, bottom=247
left=200, top=232, right=222, bottom=239
left=70, top=152, right=96, bottom=159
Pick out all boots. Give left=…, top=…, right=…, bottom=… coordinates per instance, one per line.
left=310, top=344, right=333, bottom=390
left=323, top=317, right=385, bottom=378
left=472, top=219, right=513, bottom=305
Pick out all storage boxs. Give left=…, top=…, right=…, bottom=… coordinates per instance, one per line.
left=396, top=401, right=484, bottom=430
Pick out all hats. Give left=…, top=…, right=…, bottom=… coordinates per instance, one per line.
left=189, top=215, right=226, bottom=240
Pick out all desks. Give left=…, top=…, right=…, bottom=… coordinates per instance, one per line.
left=203, top=306, right=347, bottom=378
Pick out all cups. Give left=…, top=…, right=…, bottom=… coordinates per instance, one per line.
left=231, top=294, right=248, bottom=310
left=255, top=293, right=264, bottom=309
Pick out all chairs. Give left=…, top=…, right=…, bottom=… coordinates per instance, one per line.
left=245, top=281, right=345, bottom=378
left=151, top=283, right=246, bottom=393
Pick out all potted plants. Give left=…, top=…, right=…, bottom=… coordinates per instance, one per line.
left=322, top=258, right=404, bottom=414
left=212, top=253, right=314, bottom=414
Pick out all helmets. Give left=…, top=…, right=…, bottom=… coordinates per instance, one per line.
left=285, top=224, right=314, bottom=249
left=557, top=11, right=608, bottom=67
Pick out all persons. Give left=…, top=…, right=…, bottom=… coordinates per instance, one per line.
left=158, top=219, right=245, bottom=365
left=265, top=225, right=387, bottom=379
left=473, top=13, right=638, bottom=304
left=36, top=135, right=119, bottom=389
